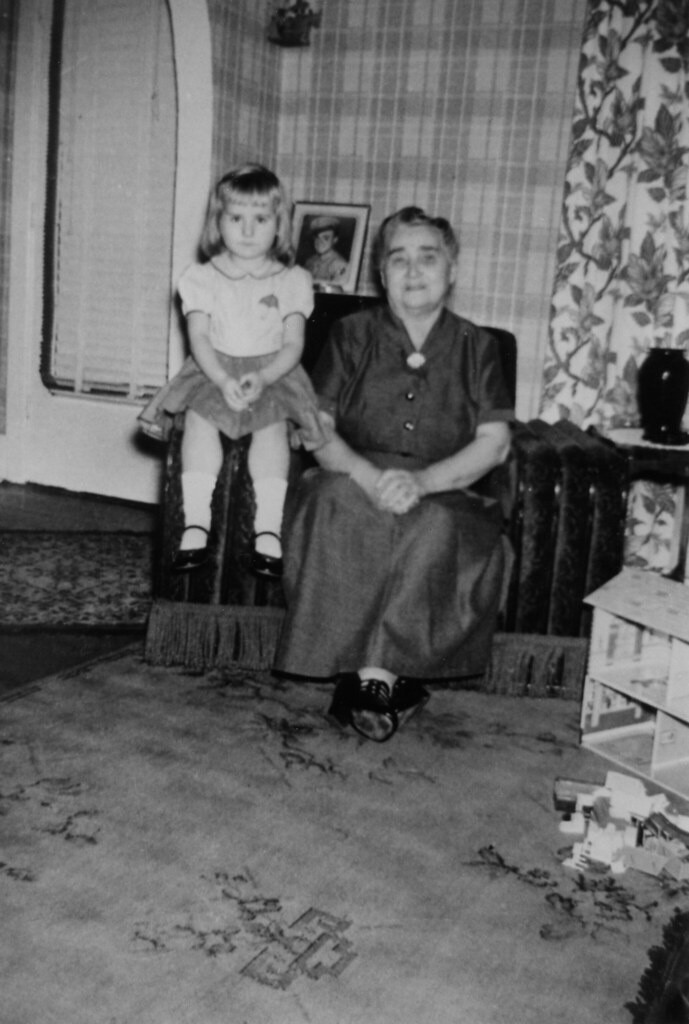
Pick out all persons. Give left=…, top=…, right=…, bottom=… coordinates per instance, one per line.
left=135, top=161, right=323, bottom=576
left=305, top=217, right=349, bottom=280
left=274, top=205, right=511, bottom=740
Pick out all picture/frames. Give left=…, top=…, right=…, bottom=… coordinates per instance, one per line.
left=287, top=201, right=371, bottom=295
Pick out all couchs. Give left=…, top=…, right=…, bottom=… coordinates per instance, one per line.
left=151, top=417, right=623, bottom=697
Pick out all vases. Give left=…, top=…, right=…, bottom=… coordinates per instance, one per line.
left=635, top=345, right=689, bottom=440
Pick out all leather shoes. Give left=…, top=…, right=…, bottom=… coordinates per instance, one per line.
left=173, top=525, right=212, bottom=573
left=249, top=531, right=285, bottom=577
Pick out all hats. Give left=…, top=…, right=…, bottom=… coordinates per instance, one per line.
left=307, top=216, right=342, bottom=233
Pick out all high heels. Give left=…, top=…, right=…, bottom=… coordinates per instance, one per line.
left=328, top=670, right=430, bottom=742
